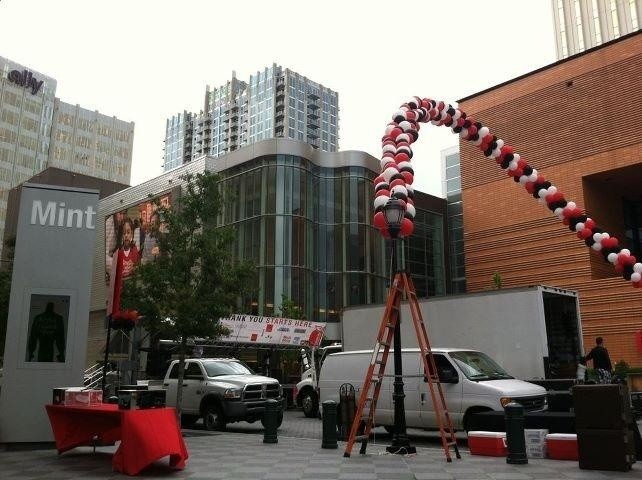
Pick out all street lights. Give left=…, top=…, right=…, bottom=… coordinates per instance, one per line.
left=381, top=188, right=407, bottom=434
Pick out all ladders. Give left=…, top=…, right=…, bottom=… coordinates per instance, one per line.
left=343, top=267, right=464, bottom=462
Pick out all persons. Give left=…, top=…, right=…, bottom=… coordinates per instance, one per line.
left=105, top=220, right=141, bottom=318
left=104, top=210, right=128, bottom=277
left=140, top=211, right=165, bottom=266
left=132, top=217, right=141, bottom=255
left=140, top=217, right=147, bottom=251
left=582, top=336, right=612, bottom=385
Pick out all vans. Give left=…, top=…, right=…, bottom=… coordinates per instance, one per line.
left=318, top=347, right=551, bottom=438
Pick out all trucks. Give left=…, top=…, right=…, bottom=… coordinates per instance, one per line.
left=293, top=283, right=585, bottom=419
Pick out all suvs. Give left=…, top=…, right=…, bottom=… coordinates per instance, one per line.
left=161, top=356, right=284, bottom=431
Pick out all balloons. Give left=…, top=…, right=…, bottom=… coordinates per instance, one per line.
left=370, top=95, right=641, bottom=291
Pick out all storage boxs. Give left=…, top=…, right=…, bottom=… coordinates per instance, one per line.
left=468, top=431, right=507, bottom=457
left=546, top=433, right=579, bottom=461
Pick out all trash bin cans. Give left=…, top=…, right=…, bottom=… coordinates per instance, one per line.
left=572, top=383, right=629, bottom=472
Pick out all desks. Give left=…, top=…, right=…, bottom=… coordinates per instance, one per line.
left=46, top=403, right=177, bottom=475
